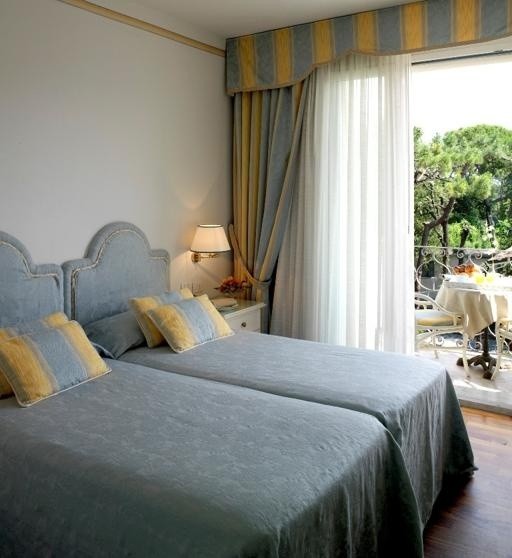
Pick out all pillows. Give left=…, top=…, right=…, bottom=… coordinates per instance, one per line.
left=0, top=287, right=236, bottom=409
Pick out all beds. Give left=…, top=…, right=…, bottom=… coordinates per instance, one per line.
left=61, top=221, right=478, bottom=533
left=0, top=230, right=425, bottom=558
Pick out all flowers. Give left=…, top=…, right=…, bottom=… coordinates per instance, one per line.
left=214, top=276, right=245, bottom=297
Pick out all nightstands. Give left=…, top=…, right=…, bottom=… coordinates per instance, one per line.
left=219, top=299, right=267, bottom=333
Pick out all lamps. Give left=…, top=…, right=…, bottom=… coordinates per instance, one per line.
left=189, top=224, right=231, bottom=263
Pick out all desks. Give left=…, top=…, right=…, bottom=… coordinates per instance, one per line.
left=433, top=273, right=512, bottom=379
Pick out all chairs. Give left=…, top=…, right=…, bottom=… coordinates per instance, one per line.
left=415, top=292, right=471, bottom=378
left=490, top=318, right=512, bottom=381
